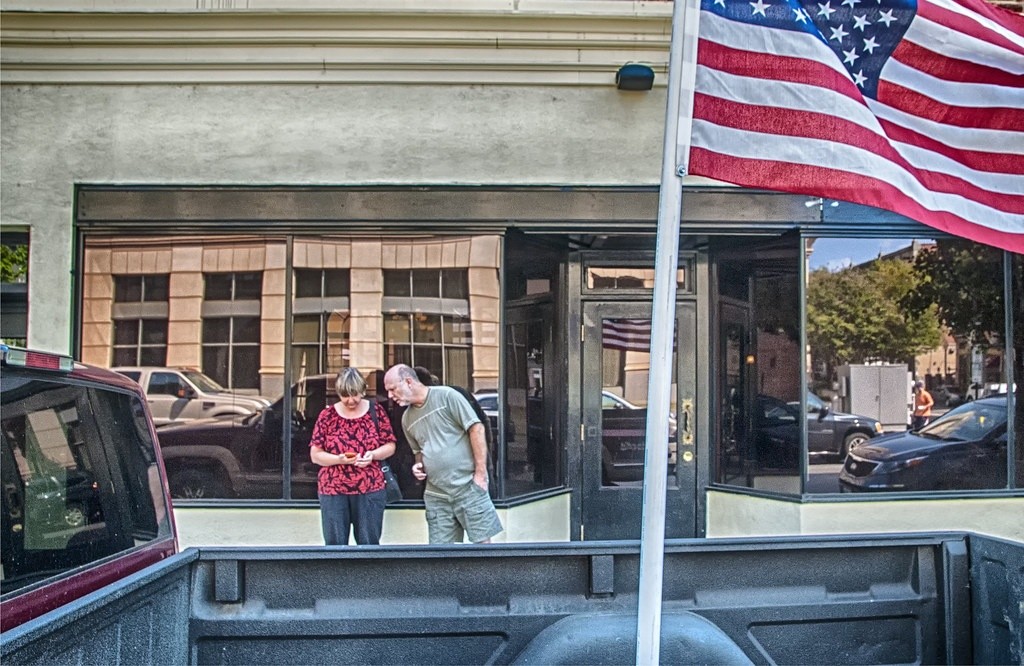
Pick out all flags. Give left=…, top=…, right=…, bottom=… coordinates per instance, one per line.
left=680, top=1, right=1023, bottom=258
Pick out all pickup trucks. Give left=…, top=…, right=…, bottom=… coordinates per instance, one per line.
left=148, top=368, right=651, bottom=511
left=1, top=338, right=1024, bottom=666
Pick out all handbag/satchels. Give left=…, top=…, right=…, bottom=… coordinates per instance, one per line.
left=380, top=459, right=403, bottom=503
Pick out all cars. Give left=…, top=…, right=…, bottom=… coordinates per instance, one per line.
left=728, top=386, right=800, bottom=462
left=933, top=385, right=962, bottom=407
left=105, top=361, right=264, bottom=437
left=594, top=389, right=654, bottom=415
left=473, top=379, right=502, bottom=426
left=965, top=381, right=987, bottom=402
left=805, top=383, right=883, bottom=459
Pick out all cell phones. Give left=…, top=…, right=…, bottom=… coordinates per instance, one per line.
left=345, top=452, right=356, bottom=458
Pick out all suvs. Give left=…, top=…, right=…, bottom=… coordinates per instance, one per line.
left=835, top=393, right=1024, bottom=492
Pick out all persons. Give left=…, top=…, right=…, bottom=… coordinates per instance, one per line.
left=911, top=381, right=933, bottom=432
left=383, top=364, right=504, bottom=546
left=310, top=365, right=397, bottom=547
